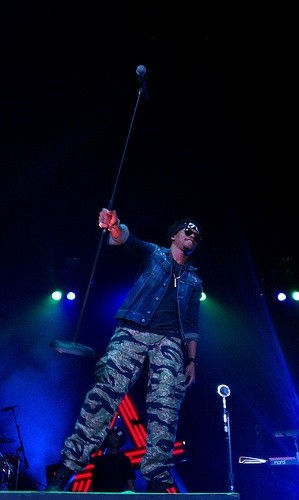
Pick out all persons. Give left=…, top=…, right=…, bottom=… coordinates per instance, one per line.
left=93, top=427, right=136, bottom=492
left=46, top=206, right=204, bottom=494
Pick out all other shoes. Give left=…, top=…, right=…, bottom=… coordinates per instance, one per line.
left=44, top=462, right=75, bottom=493
left=146, top=481, right=174, bottom=493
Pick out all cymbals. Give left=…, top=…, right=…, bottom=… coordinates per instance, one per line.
left=0, top=437, right=17, bottom=445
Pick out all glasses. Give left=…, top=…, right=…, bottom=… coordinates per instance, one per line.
left=180, top=227, right=202, bottom=243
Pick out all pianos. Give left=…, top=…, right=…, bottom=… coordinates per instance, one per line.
left=268, top=456, right=298, bottom=467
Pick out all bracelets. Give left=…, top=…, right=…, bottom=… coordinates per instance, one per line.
left=188, top=357, right=196, bottom=363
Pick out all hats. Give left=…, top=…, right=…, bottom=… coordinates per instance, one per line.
left=169, top=219, right=202, bottom=237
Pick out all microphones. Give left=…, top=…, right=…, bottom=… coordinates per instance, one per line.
left=2, top=406, right=15, bottom=412
left=135, top=64, right=147, bottom=82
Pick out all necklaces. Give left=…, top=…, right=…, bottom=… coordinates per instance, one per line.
left=172, top=268, right=184, bottom=287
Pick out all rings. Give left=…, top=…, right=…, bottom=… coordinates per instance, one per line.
left=98, top=221, right=103, bottom=227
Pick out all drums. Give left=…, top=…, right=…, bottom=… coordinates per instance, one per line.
left=1, top=454, right=20, bottom=475
